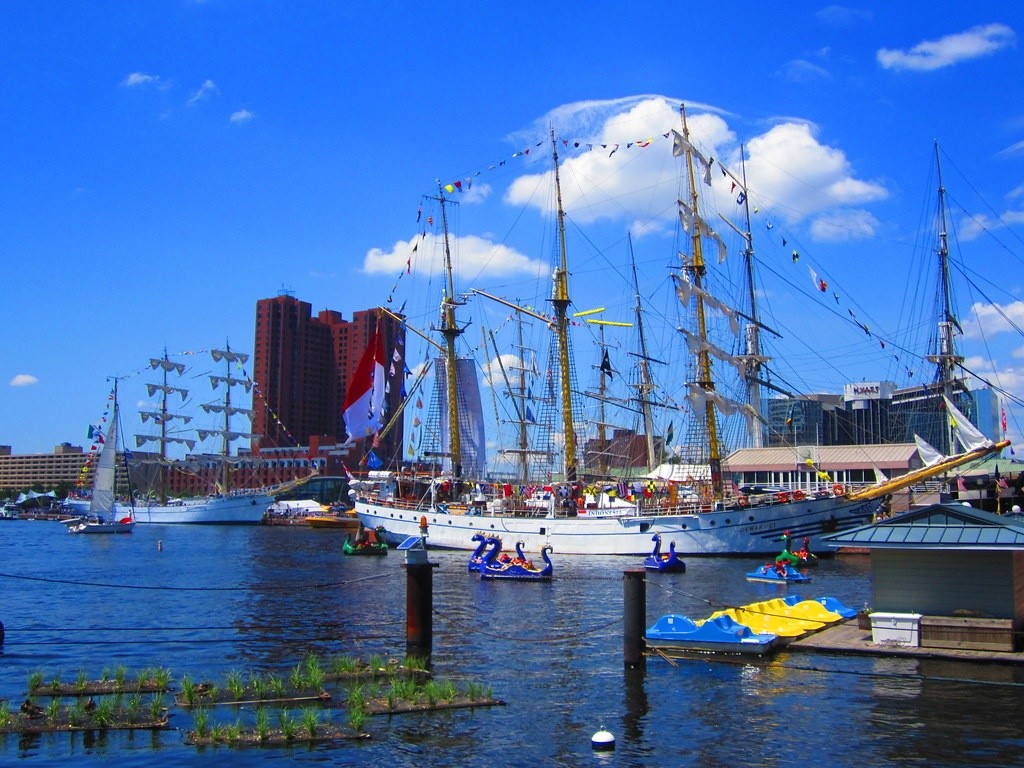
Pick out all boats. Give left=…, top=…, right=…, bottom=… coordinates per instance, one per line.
left=776, top=526, right=818, bottom=567
left=307, top=500, right=362, bottom=529
left=353, top=100, right=1015, bottom=559
left=640, top=591, right=858, bottom=655
left=641, top=532, right=687, bottom=572
left=69, top=518, right=136, bottom=535
left=341, top=524, right=387, bottom=556
left=468, top=531, right=556, bottom=582
left=746, top=556, right=815, bottom=584
left=259, top=506, right=318, bottom=525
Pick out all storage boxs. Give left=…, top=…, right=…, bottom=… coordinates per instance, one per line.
left=867, top=612, right=924, bottom=646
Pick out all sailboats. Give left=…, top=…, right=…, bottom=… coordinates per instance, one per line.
left=61, top=339, right=268, bottom=524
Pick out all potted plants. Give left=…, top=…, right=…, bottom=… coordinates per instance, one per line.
left=857, top=602, right=872, bottom=630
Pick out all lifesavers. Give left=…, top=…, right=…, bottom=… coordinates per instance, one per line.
left=738, top=497, right=747, bottom=506
left=834, top=486, right=843, bottom=495
left=778, top=492, right=788, bottom=502
left=793, top=491, right=803, bottom=500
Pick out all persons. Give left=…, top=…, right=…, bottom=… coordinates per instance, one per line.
left=128, top=510, right=132, bottom=518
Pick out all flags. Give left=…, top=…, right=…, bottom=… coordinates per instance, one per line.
left=1001, top=407, right=1006, bottom=431
left=75, top=389, right=115, bottom=489
left=122, top=130, right=992, bottom=519
